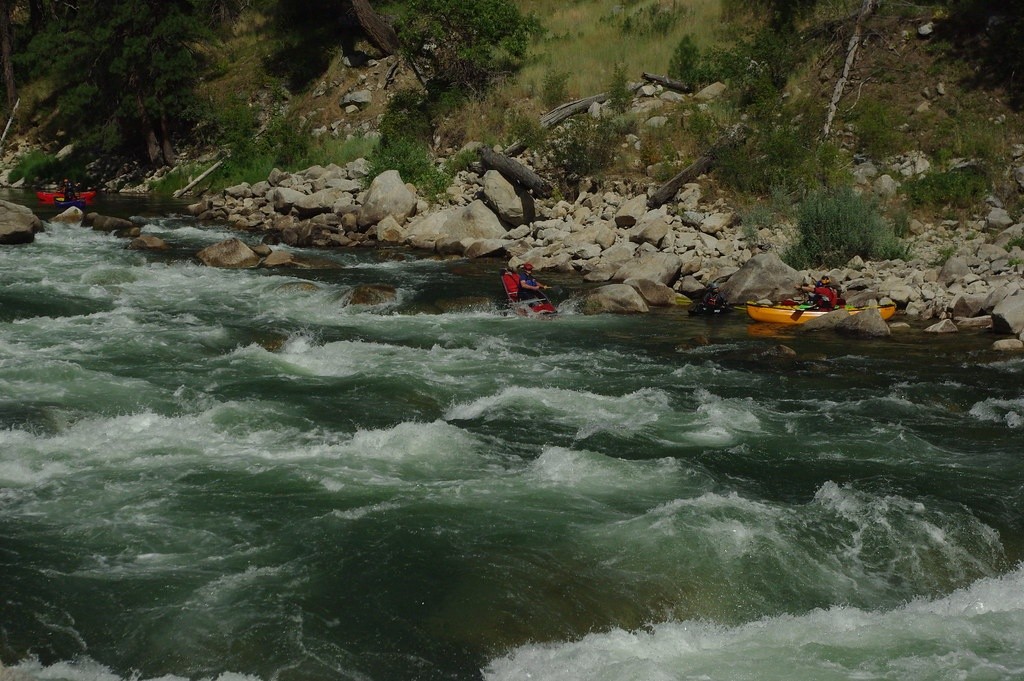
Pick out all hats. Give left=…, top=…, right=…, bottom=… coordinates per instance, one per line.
left=524, top=263, right=534, bottom=270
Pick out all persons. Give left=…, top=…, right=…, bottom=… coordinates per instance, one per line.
left=702, top=286, right=723, bottom=312
left=517, top=264, right=547, bottom=307
left=794, top=276, right=837, bottom=310
left=62, top=179, right=76, bottom=202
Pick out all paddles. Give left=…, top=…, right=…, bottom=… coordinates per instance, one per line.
left=539, top=286, right=553, bottom=289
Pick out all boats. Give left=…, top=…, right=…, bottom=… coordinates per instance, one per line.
left=54, top=195, right=87, bottom=207
left=744, top=301, right=897, bottom=325
left=497, top=267, right=559, bottom=318
left=38, top=190, right=97, bottom=202
